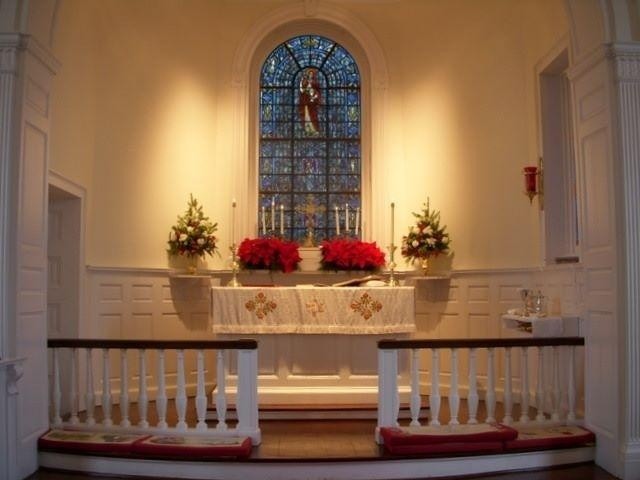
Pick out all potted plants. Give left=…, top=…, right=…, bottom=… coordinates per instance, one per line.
left=170, top=198, right=449, bottom=276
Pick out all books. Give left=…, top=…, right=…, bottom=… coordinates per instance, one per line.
left=330, top=274, right=382, bottom=288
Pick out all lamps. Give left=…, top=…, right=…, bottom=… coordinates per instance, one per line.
left=522, top=154, right=544, bottom=206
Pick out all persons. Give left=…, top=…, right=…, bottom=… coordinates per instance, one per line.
left=298, top=69, right=324, bottom=138
